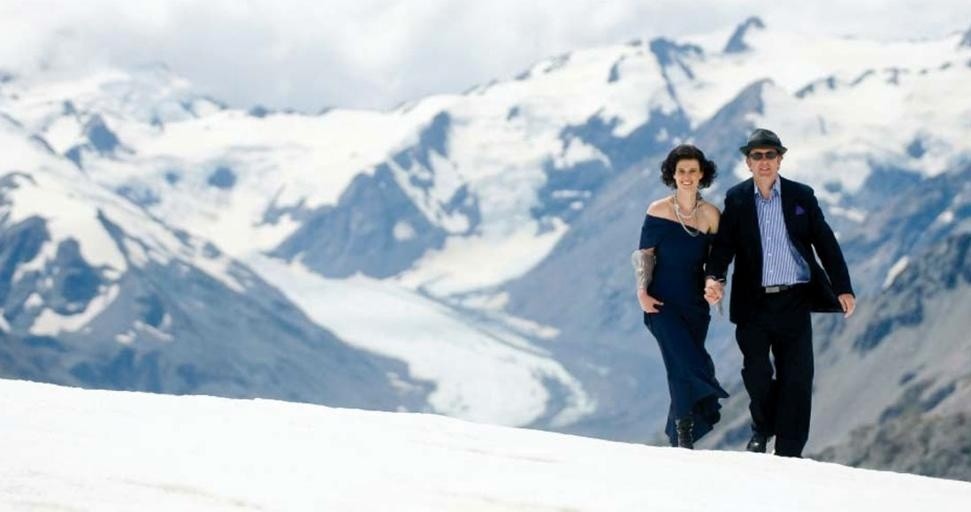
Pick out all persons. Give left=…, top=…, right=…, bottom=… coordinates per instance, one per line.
left=631, top=145, right=730, bottom=451
left=705, top=128, right=856, bottom=459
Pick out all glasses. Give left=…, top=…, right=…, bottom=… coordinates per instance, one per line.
left=747, top=151, right=780, bottom=159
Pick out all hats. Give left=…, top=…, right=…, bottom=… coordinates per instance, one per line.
left=739, top=128, right=788, bottom=156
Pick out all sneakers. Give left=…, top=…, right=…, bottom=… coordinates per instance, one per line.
left=747, top=434, right=773, bottom=453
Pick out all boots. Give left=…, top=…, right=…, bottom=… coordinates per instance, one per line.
left=675, top=411, right=694, bottom=450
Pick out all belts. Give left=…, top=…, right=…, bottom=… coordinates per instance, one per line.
left=762, top=283, right=805, bottom=294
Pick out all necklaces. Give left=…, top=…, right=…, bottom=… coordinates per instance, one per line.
left=672, top=191, right=701, bottom=238
